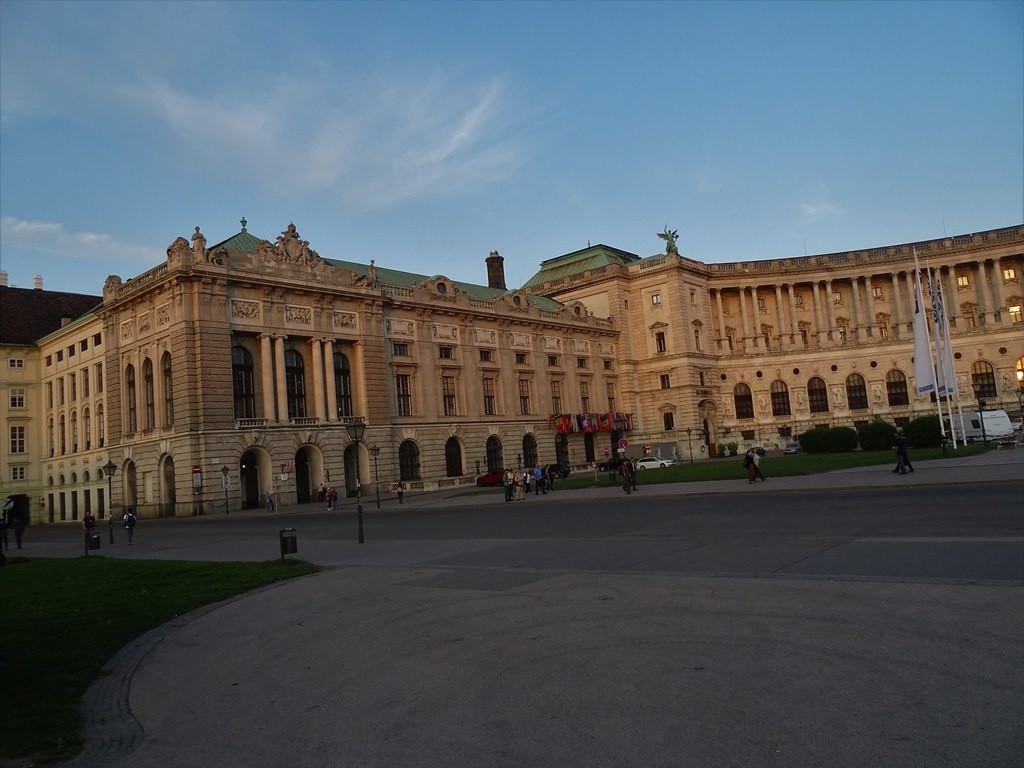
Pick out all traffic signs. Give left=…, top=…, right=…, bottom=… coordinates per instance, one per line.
left=618, top=448, right=625, bottom=453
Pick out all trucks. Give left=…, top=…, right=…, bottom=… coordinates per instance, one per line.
left=945, top=410, right=1015, bottom=442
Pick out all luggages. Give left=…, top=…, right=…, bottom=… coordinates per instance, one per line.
left=88, top=531, right=100, bottom=550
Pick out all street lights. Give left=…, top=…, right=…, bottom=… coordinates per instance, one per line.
left=345, top=419, right=366, bottom=543
left=516, top=453, right=523, bottom=471
left=1015, top=387, right=1023, bottom=419
left=220, top=464, right=229, bottom=515
left=370, top=444, right=381, bottom=508
left=971, top=378, right=988, bottom=445
left=101, top=457, right=118, bottom=544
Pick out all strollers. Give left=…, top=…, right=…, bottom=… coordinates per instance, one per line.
left=85, top=527, right=101, bottom=550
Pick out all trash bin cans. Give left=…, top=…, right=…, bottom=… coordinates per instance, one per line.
left=86, top=531, right=101, bottom=550
left=280, top=528, right=298, bottom=553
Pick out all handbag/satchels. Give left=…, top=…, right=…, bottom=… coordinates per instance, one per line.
left=619, top=467, right=622, bottom=475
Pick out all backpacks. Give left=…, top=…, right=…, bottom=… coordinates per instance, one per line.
left=744, top=462, right=748, bottom=468
left=126, top=514, right=136, bottom=527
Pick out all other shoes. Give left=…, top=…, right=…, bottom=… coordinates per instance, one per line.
left=899, top=473, right=907, bottom=474
left=506, top=499, right=509, bottom=502
left=750, top=481, right=756, bottom=484
left=623, top=487, right=627, bottom=492
left=754, top=481, right=757, bottom=482
left=892, top=470, right=898, bottom=472
left=633, top=489, right=638, bottom=491
left=520, top=498, right=525, bottom=500
left=627, top=491, right=630, bottom=495
left=509, top=499, right=514, bottom=501
left=515, top=499, right=520, bottom=501
left=536, top=494, right=539, bottom=495
left=128, top=542, right=133, bottom=545
left=761, top=479, right=767, bottom=481
left=909, top=470, right=913, bottom=472
left=543, top=492, right=547, bottom=494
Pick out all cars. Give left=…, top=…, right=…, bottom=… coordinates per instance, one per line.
left=477, top=469, right=505, bottom=487
left=783, top=443, right=800, bottom=455
left=635, top=456, right=676, bottom=470
left=1010, top=417, right=1024, bottom=429
left=598, top=458, right=625, bottom=472
left=748, top=447, right=767, bottom=458
left=530, top=463, right=571, bottom=480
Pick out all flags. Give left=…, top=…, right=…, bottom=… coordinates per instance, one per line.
left=927, top=273, right=948, bottom=396
left=937, top=275, right=956, bottom=395
left=914, top=266, right=936, bottom=395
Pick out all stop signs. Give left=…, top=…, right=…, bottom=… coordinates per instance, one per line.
left=604, top=447, right=608, bottom=453
left=191, top=465, right=202, bottom=473
left=644, top=444, right=651, bottom=449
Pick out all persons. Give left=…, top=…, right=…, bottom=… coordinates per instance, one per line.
left=892, top=433, right=914, bottom=475
left=534, top=464, right=547, bottom=495
left=621, top=457, right=639, bottom=493
left=318, top=483, right=338, bottom=511
left=0, top=518, right=8, bottom=551
left=664, top=226, right=679, bottom=251
left=356, top=478, right=361, bottom=497
left=503, top=468, right=525, bottom=502
left=524, top=468, right=533, bottom=497
left=264, top=491, right=274, bottom=512
left=397, top=482, right=403, bottom=504
left=123, top=508, right=136, bottom=545
left=192, top=226, right=205, bottom=261
left=545, top=464, right=555, bottom=492
left=83, top=511, right=96, bottom=547
left=744, top=448, right=766, bottom=483
left=14, top=518, right=25, bottom=549
left=369, top=260, right=378, bottom=289
left=274, top=232, right=312, bottom=265
